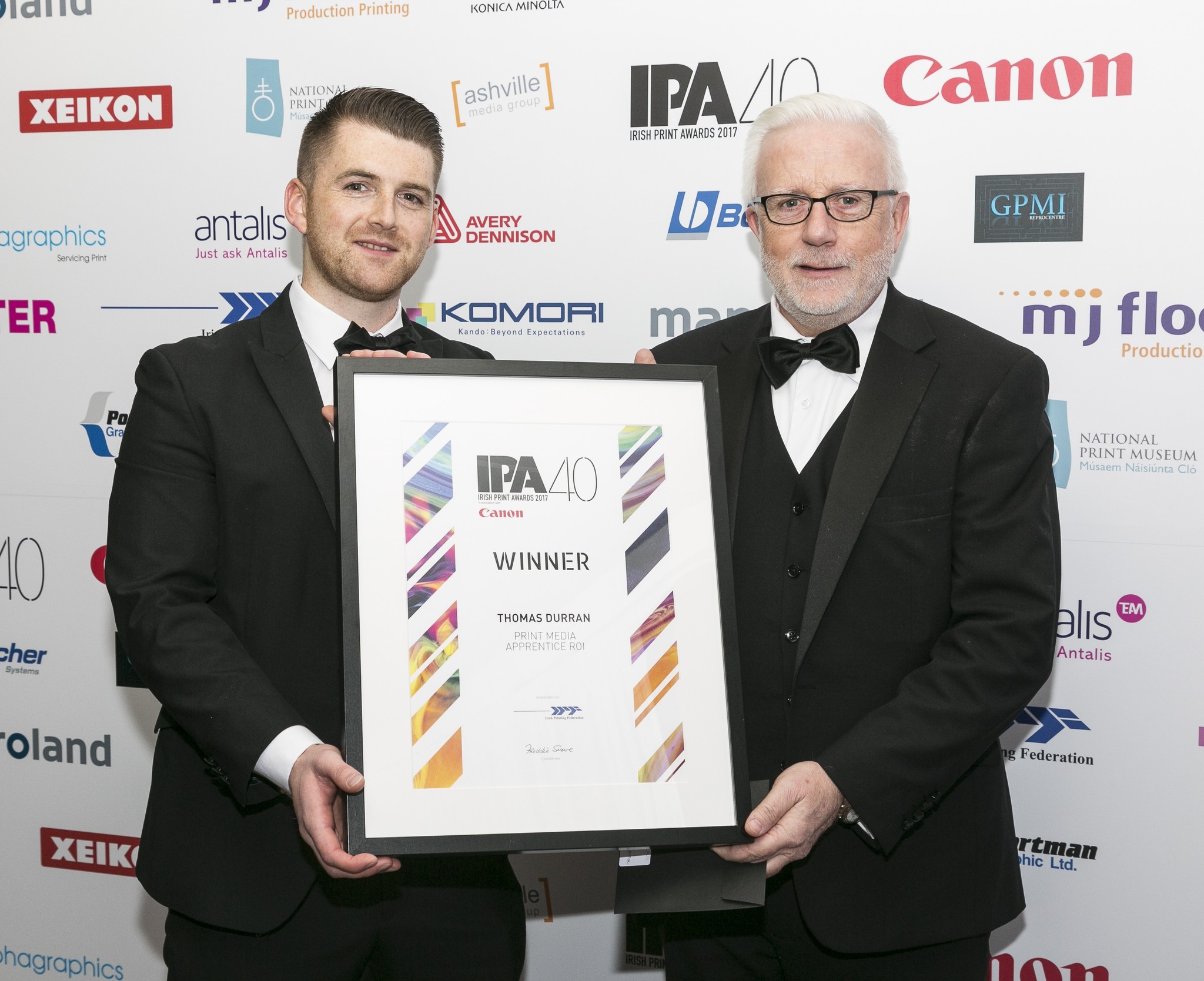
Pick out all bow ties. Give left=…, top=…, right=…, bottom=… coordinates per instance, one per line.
left=333, top=320, right=418, bottom=356
left=756, top=323, right=860, bottom=390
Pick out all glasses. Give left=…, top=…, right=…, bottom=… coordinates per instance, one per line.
left=751, top=190, right=898, bottom=225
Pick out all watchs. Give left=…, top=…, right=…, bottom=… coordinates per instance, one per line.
left=838, top=791, right=863, bottom=824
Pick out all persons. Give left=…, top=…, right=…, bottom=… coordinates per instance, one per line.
left=103, top=86, right=529, bottom=981
left=619, top=95, right=1061, bottom=981
